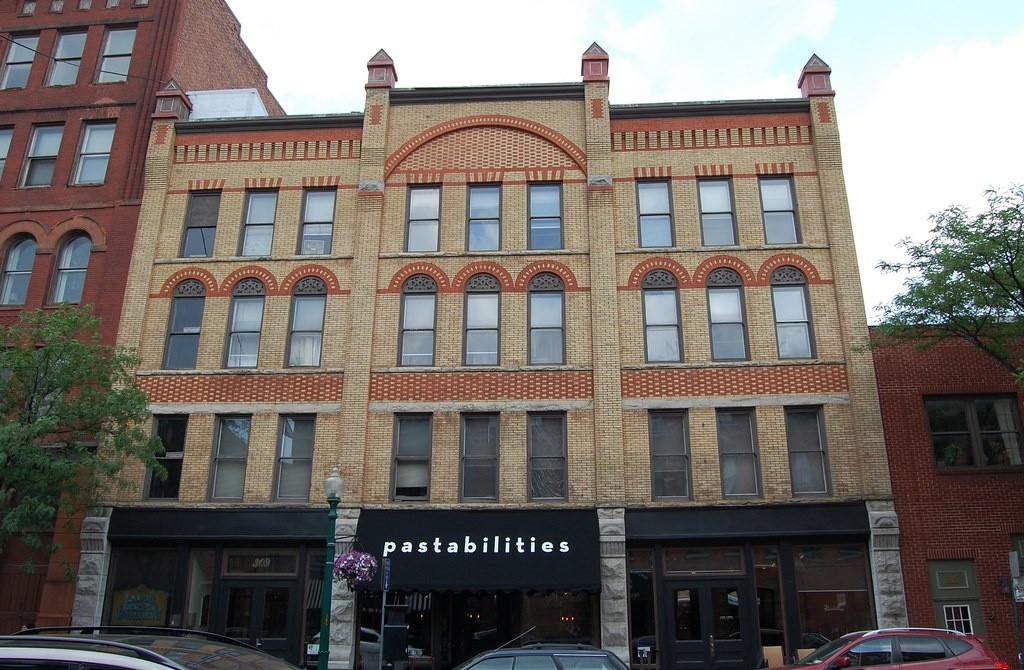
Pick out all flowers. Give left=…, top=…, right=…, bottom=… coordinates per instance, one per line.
left=334, top=550, right=377, bottom=591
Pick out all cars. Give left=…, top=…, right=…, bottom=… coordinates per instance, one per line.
left=630, top=635, right=657, bottom=665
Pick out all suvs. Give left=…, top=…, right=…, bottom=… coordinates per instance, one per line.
left=0, top=624, right=304, bottom=670
left=453, top=642, right=631, bottom=670
left=764, top=627, right=1012, bottom=670
left=310, top=626, right=423, bottom=670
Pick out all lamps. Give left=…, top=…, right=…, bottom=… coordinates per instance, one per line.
left=1001, top=576, right=1010, bottom=595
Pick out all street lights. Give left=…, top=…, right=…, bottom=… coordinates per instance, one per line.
left=318, top=464, right=346, bottom=670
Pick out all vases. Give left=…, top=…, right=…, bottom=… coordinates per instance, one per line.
left=347, top=578, right=364, bottom=591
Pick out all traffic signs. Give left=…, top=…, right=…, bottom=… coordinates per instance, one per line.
left=383, top=559, right=391, bottom=592
